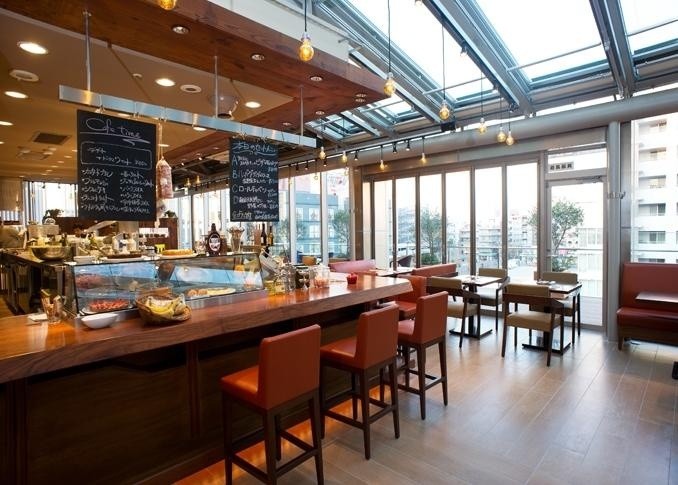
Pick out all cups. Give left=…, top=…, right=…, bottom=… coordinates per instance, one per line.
left=40, top=289, right=67, bottom=325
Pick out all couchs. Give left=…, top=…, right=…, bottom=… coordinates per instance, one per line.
left=329, top=258, right=376, bottom=270
left=411, top=262, right=457, bottom=284
left=619, top=261, right=678, bottom=350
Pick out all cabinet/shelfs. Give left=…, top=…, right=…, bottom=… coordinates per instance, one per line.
left=61, top=253, right=263, bottom=320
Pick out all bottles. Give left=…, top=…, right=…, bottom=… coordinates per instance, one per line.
left=267, top=225, right=274, bottom=246
left=260, top=223, right=267, bottom=246
left=59, top=234, right=65, bottom=246
left=207, top=223, right=222, bottom=256
left=295, top=267, right=310, bottom=291
left=128, top=233, right=136, bottom=251
left=63, top=232, right=68, bottom=247
left=119, top=232, right=127, bottom=251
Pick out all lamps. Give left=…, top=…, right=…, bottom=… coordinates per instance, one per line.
left=420, top=135, right=429, bottom=163
left=322, top=157, right=327, bottom=168
left=341, top=117, right=347, bottom=163
left=497, top=88, right=506, bottom=142
left=379, top=144, right=386, bottom=169
left=353, top=149, right=359, bottom=160
left=378, top=287, right=450, bottom=421
left=319, top=119, right=326, bottom=159
left=297, top=0, right=314, bottom=60
left=391, top=140, right=397, bottom=153
left=507, top=104, right=515, bottom=144
left=405, top=139, right=411, bottom=151
left=318, top=303, right=400, bottom=461
left=295, top=162, right=299, bottom=172
left=477, top=62, right=489, bottom=132
left=440, top=18, right=450, bottom=119
left=383, top=0, right=396, bottom=95
left=304, top=160, right=309, bottom=171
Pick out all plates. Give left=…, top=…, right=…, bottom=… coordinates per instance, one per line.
left=72, top=255, right=95, bottom=264
left=158, top=254, right=197, bottom=260
left=99, top=257, right=145, bottom=262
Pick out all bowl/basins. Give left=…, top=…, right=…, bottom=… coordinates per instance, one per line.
left=80, top=312, right=118, bottom=329
left=30, top=246, right=71, bottom=261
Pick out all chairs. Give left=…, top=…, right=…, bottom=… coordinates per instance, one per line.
left=388, top=272, right=428, bottom=380
left=222, top=324, right=324, bottom=485
left=476, top=267, right=512, bottom=331
left=428, top=276, right=480, bottom=346
left=499, top=284, right=567, bottom=367
left=389, top=255, right=412, bottom=266
left=535, top=271, right=582, bottom=338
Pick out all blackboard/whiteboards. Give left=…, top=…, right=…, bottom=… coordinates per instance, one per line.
left=77, top=110, right=156, bottom=220
left=230, top=138, right=279, bottom=222
